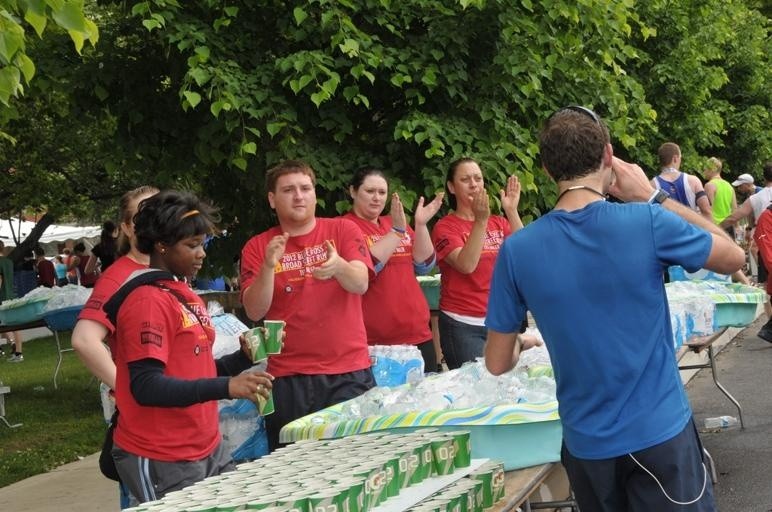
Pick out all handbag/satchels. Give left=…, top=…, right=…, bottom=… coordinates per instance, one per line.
left=100, top=406, right=121, bottom=482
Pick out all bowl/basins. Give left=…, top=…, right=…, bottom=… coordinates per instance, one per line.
left=706, top=283, right=769, bottom=328
left=0, top=294, right=86, bottom=332
left=415, top=278, right=445, bottom=311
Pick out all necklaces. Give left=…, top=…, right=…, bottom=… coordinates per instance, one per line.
left=555, top=185, right=607, bottom=204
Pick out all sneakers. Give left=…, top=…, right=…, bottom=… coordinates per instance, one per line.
left=6, top=339, right=24, bottom=362
left=757, top=325, right=772, bottom=342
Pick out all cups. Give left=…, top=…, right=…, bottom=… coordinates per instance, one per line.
left=405, top=462, right=508, bottom=511
left=252, top=381, right=275, bottom=417
left=123, top=427, right=470, bottom=512
left=244, top=327, right=268, bottom=364
left=262, top=320, right=284, bottom=354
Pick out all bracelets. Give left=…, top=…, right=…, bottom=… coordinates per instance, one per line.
left=517, top=335, right=523, bottom=353
left=391, top=228, right=404, bottom=238
left=392, top=226, right=405, bottom=233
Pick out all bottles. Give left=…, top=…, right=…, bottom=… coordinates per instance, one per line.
left=661, top=281, right=719, bottom=352
left=704, top=415, right=736, bottom=429
left=735, top=224, right=744, bottom=241
left=331, top=341, right=556, bottom=422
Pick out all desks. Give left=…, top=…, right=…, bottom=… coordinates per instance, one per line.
left=484, top=346, right=717, bottom=511
left=682, top=327, right=745, bottom=433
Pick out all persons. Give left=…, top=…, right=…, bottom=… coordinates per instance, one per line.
left=431, top=157, right=525, bottom=371
left=649, top=141, right=772, bottom=343
left=484, top=105, right=746, bottom=512
left=237, top=160, right=377, bottom=453
left=334, top=165, right=446, bottom=373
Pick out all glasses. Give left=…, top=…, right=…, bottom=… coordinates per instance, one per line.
left=546, top=105, right=606, bottom=147
